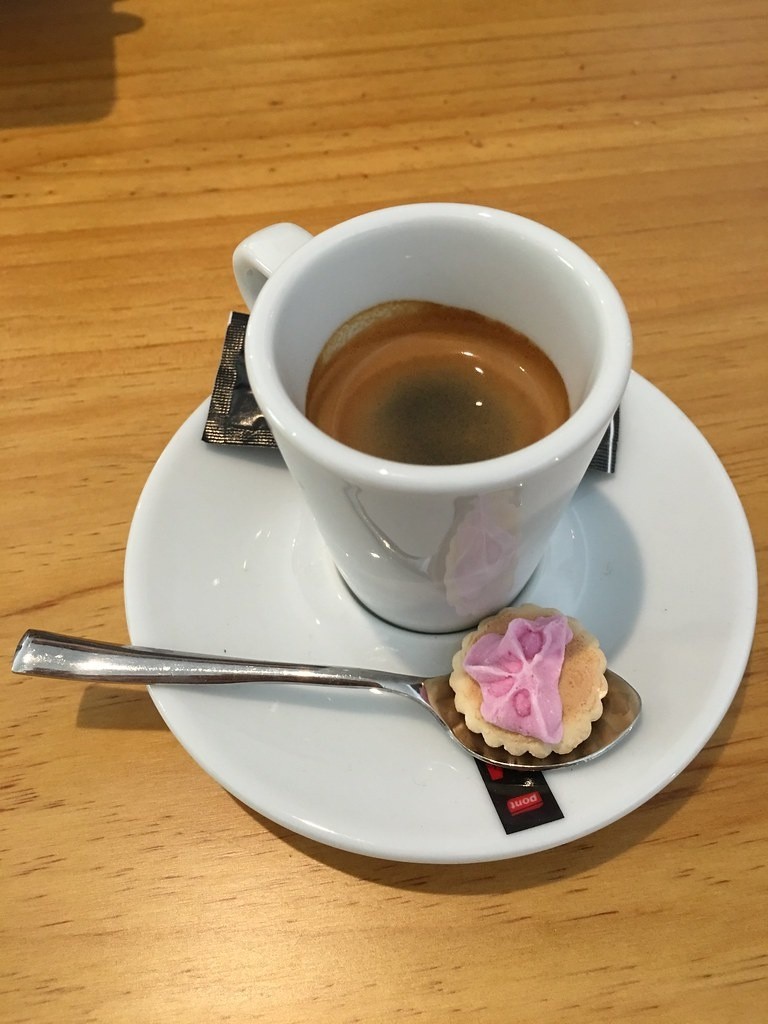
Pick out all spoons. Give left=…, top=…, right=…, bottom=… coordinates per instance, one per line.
left=8, top=628, right=646, bottom=768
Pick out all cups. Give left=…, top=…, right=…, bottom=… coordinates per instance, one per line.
left=230, top=201, right=630, bottom=635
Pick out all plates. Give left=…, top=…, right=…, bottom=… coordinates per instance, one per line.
left=124, top=372, right=759, bottom=865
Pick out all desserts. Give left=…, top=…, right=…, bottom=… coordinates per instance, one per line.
left=452, top=606, right=609, bottom=761
